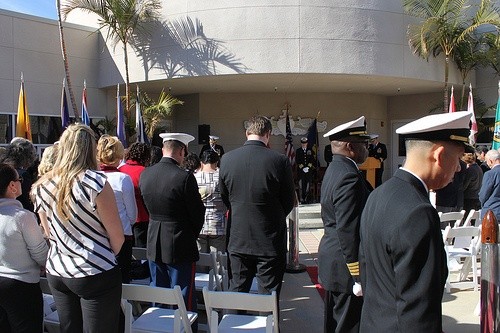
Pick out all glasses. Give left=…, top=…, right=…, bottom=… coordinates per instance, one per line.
left=15, top=176, right=24, bottom=183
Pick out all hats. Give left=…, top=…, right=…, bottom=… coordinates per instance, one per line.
left=209, top=135, right=219, bottom=141
left=300, top=137, right=308, bottom=144
left=159, top=133, right=195, bottom=156
left=370, top=135, right=379, bottom=142
left=323, top=115, right=371, bottom=141
left=396, top=111, right=475, bottom=153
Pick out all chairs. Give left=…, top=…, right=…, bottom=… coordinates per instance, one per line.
left=438, top=208, right=480, bottom=297
left=40, top=244, right=278, bottom=333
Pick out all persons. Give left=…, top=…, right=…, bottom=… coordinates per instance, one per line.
left=296, top=138, right=315, bottom=204
left=318, top=111, right=500, bottom=333
left=0, top=123, right=224, bottom=333
left=219, top=114, right=295, bottom=318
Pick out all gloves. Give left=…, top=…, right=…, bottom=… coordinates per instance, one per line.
left=353, top=282, right=363, bottom=296
left=303, top=167, right=309, bottom=173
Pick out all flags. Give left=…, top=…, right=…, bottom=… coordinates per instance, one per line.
left=82, top=88, right=91, bottom=128
left=15, top=81, right=33, bottom=143
left=284, top=114, right=296, bottom=171
left=449, top=91, right=478, bottom=147
left=491, top=95, right=500, bottom=154
left=116, top=92, right=128, bottom=147
left=136, top=93, right=150, bottom=145
left=60, top=86, right=70, bottom=136
